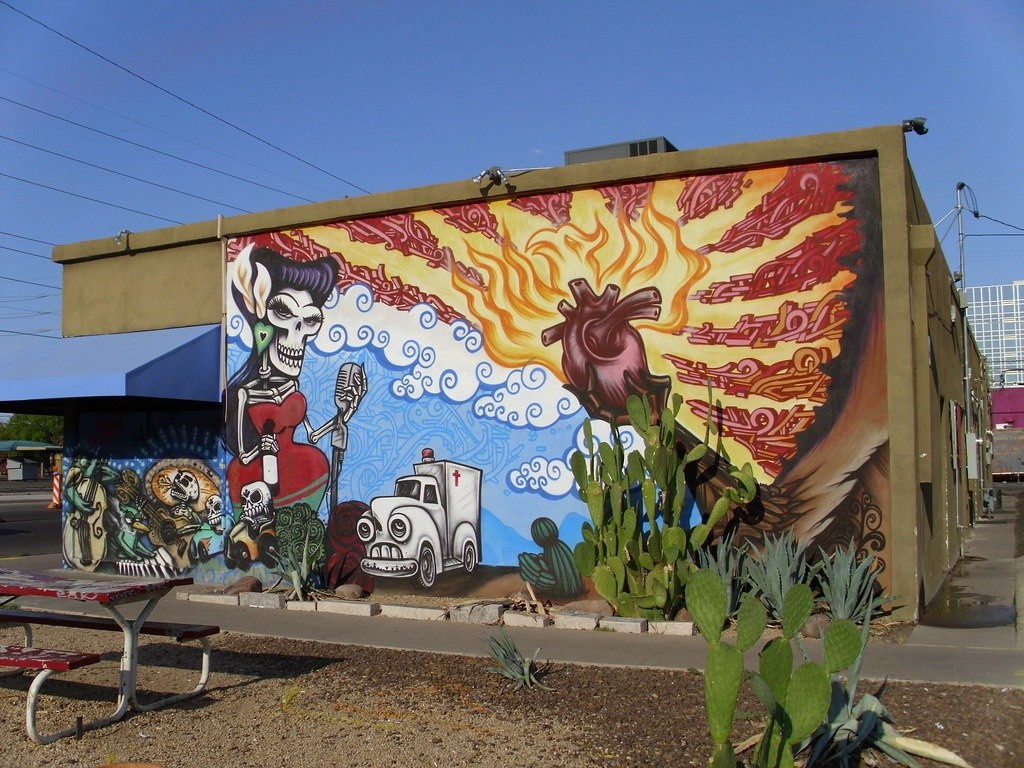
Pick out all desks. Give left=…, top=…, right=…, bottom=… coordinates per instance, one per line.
left=0, top=566, right=193, bottom=722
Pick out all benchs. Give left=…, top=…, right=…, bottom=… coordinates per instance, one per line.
left=0, top=608, right=220, bottom=745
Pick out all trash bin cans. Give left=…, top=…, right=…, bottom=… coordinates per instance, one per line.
left=6, top=457, right=39, bottom=482
